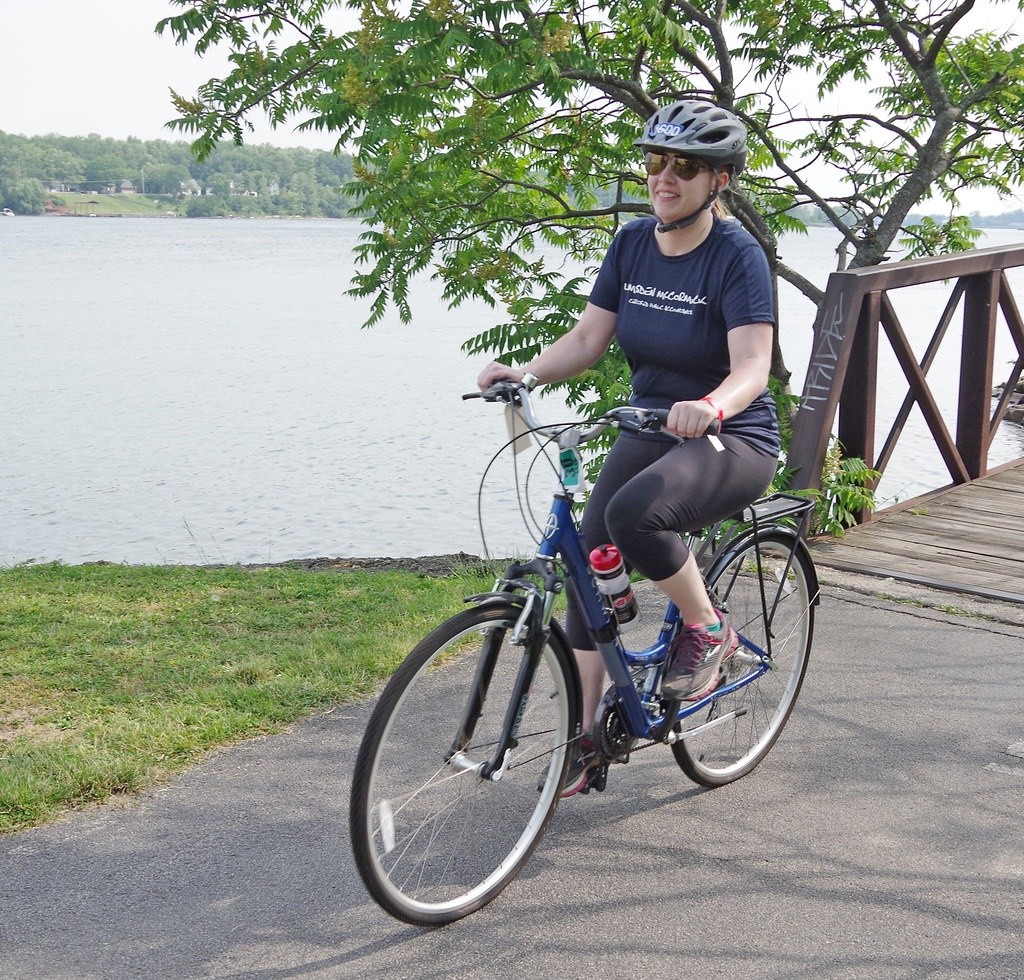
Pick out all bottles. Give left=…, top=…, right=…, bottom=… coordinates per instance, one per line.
left=590, top=542, right=639, bottom=633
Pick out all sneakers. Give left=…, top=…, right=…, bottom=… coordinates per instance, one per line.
left=660, top=609, right=738, bottom=700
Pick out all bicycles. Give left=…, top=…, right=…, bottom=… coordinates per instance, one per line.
left=348, top=363, right=826, bottom=936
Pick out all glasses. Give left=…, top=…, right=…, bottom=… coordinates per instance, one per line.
left=643, top=151, right=714, bottom=181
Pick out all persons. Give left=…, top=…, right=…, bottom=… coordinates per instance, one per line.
left=475, top=100, right=782, bottom=799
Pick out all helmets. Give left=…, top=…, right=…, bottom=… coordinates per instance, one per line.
left=537, top=722, right=603, bottom=798
left=633, top=99, right=749, bottom=174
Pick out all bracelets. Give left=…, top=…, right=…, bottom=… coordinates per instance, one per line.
left=697, top=396, right=724, bottom=422
